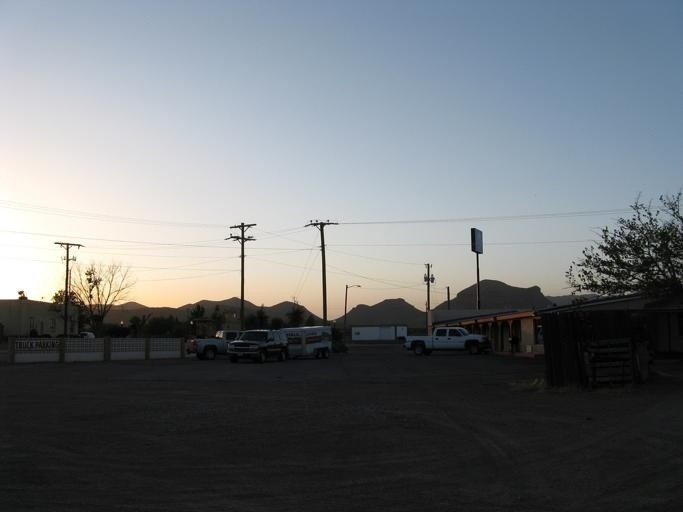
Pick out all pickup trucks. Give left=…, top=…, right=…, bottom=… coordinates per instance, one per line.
left=184, top=329, right=244, bottom=361
left=399, top=326, right=489, bottom=355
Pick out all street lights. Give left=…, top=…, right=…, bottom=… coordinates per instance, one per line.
left=344, top=285, right=361, bottom=326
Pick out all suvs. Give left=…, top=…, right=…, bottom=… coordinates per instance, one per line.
left=226, top=329, right=289, bottom=362
